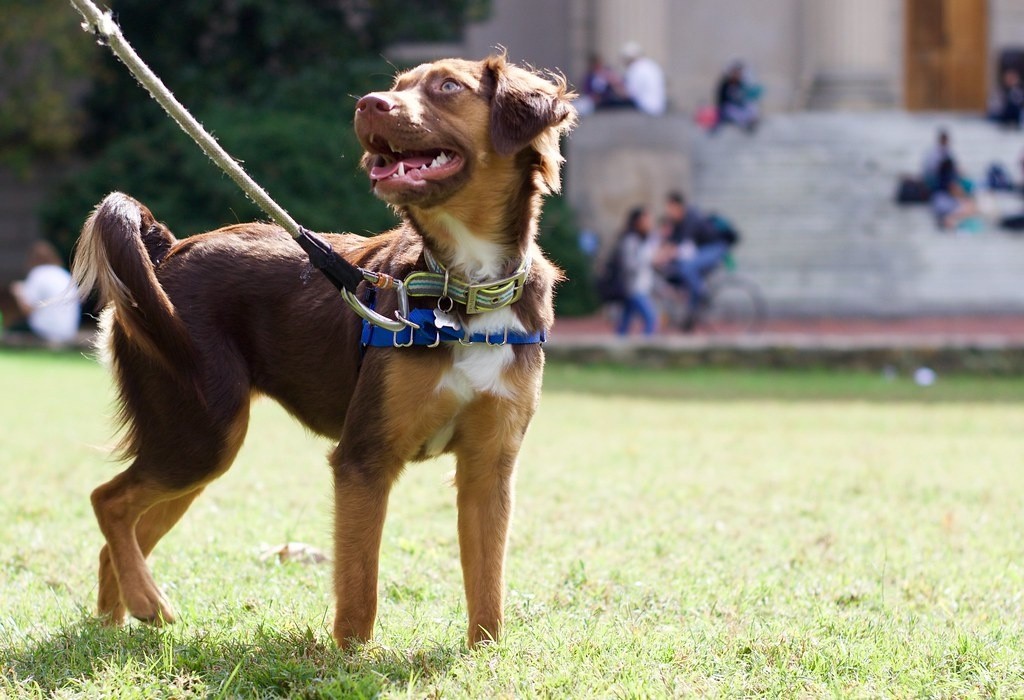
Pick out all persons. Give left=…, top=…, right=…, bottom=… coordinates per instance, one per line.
left=661, top=191, right=729, bottom=336
left=707, top=55, right=761, bottom=133
left=595, top=40, right=667, bottom=117
left=0, top=239, right=80, bottom=350
left=571, top=55, right=627, bottom=118
left=612, top=204, right=658, bottom=334
left=894, top=48, right=1023, bottom=234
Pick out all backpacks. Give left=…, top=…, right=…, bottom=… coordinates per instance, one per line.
left=596, top=234, right=638, bottom=304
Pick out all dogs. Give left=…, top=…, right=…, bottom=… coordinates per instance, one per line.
left=68, top=56, right=573, bottom=659
